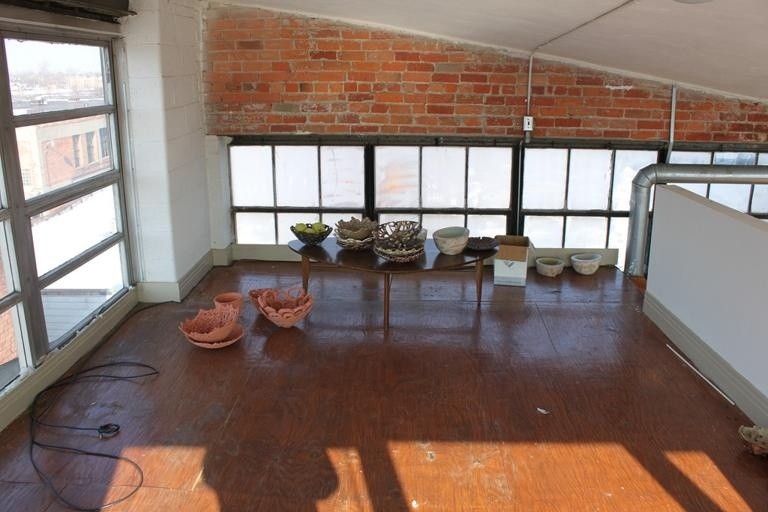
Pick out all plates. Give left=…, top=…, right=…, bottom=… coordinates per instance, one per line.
left=184, top=323, right=245, bottom=350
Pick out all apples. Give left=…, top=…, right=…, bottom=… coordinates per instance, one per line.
left=295, top=223, right=324, bottom=234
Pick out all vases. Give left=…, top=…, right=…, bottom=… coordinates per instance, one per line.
left=214, top=292, right=242, bottom=316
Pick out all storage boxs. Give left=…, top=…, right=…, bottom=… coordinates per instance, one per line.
left=494, top=235, right=536, bottom=286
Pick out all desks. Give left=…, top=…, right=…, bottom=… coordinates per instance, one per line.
left=288, top=238, right=498, bottom=332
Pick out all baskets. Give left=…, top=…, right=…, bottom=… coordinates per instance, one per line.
left=178, top=305, right=244, bottom=349
left=291, top=224, right=333, bottom=245
left=249, top=286, right=314, bottom=329
left=334, top=216, right=424, bottom=263
